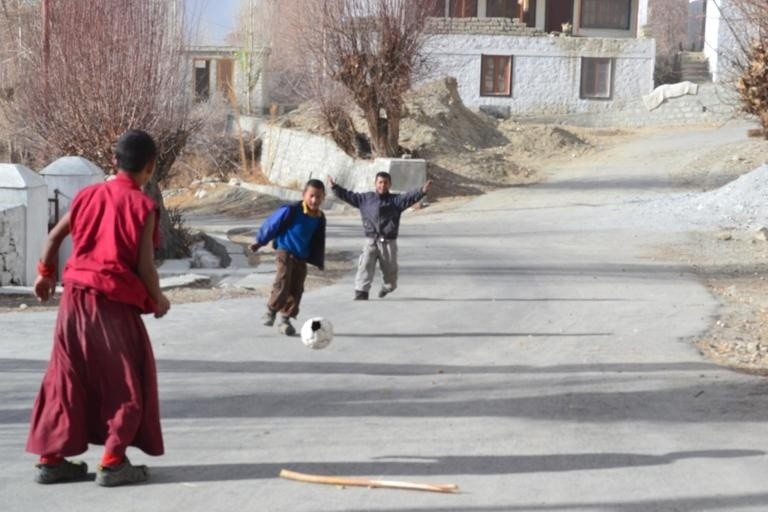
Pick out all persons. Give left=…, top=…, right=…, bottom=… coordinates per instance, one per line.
left=25, top=128, right=170, bottom=489
left=248, top=177, right=326, bottom=338
left=325, top=171, right=436, bottom=302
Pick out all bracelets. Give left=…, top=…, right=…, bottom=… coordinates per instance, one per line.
left=37, top=260, right=57, bottom=276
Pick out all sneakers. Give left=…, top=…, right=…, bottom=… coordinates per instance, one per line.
left=96, top=458, right=149, bottom=487
left=379, top=290, right=386, bottom=297
left=354, top=291, right=369, bottom=300
left=261, top=310, right=295, bottom=335
left=36, top=460, right=87, bottom=483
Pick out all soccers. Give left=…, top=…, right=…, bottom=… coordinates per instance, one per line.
left=301, top=318, right=332, bottom=349
